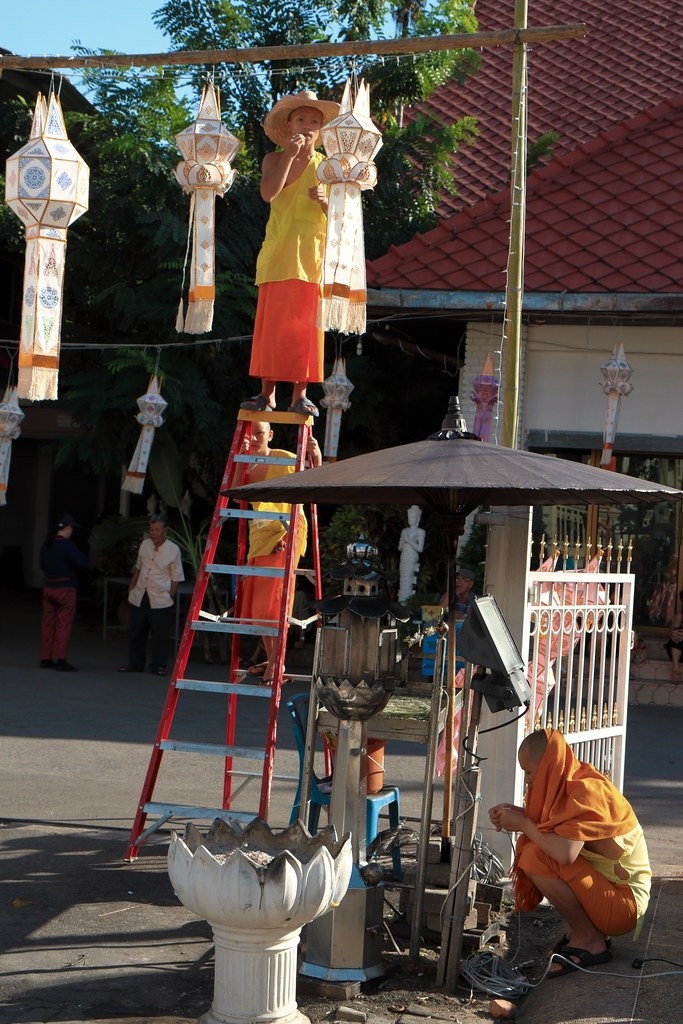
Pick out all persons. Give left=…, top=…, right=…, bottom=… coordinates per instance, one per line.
left=39, top=514, right=89, bottom=672
left=438, top=568, right=481, bottom=617
left=579, top=589, right=638, bottom=680
left=240, top=88, right=341, bottom=417
left=663, top=590, right=683, bottom=683
left=118, top=514, right=185, bottom=675
left=229, top=422, right=322, bottom=688
left=397, top=505, right=425, bottom=603
left=488, top=728, right=652, bottom=977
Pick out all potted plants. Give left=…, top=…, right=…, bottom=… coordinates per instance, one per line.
left=323, top=730, right=394, bottom=793
left=111, top=425, right=234, bottom=661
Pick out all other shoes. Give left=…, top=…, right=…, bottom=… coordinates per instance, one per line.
left=55, top=658, right=78, bottom=672
left=154, top=667, right=167, bottom=676
left=615, top=673, right=635, bottom=679
left=672, top=670, right=681, bottom=683
left=588, top=668, right=607, bottom=678
left=119, top=666, right=145, bottom=672
left=40, top=658, right=56, bottom=668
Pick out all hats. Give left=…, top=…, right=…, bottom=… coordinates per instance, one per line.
left=263, top=90, right=341, bottom=150
left=56, top=513, right=75, bottom=528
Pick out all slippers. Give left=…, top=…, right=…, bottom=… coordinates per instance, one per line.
left=288, top=398, right=319, bottom=417
left=240, top=395, right=273, bottom=412
left=246, top=662, right=270, bottom=676
left=546, top=932, right=612, bottom=961
left=260, top=677, right=288, bottom=687
left=546, top=946, right=610, bottom=979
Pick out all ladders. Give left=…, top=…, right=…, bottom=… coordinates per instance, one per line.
left=122, top=404, right=325, bottom=865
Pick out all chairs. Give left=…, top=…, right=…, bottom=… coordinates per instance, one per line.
left=286, top=692, right=401, bottom=883
